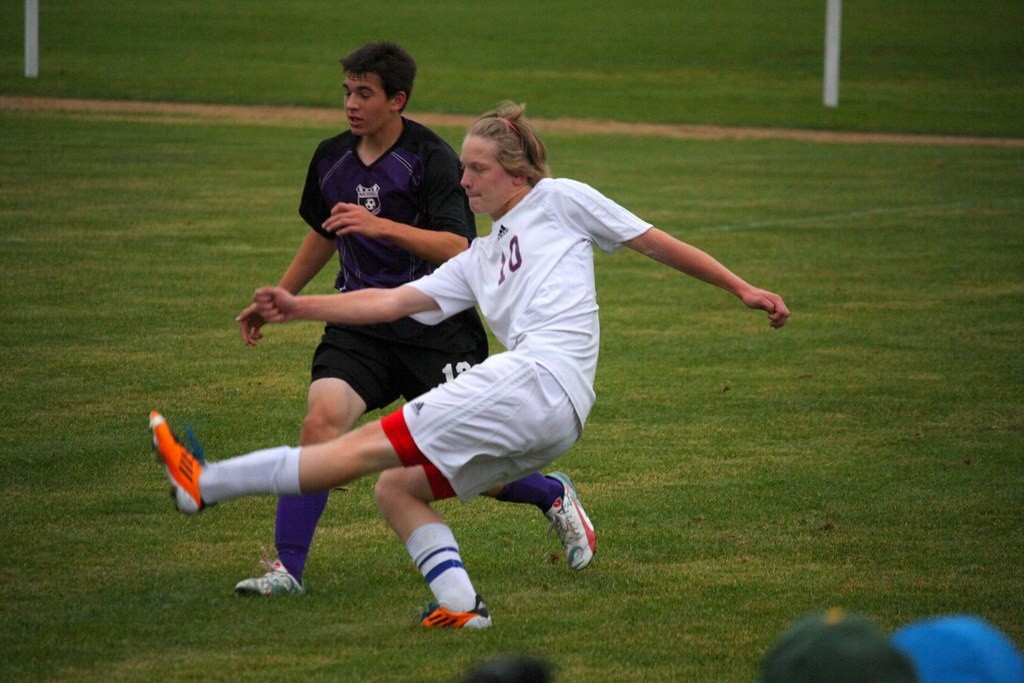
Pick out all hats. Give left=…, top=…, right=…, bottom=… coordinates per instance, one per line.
left=895, top=616, right=1024, bottom=683
left=756, top=607, right=918, bottom=683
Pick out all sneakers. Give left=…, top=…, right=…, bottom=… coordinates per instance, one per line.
left=417, top=596, right=494, bottom=629
left=233, top=560, right=306, bottom=599
left=544, top=467, right=598, bottom=571
left=149, top=409, right=218, bottom=518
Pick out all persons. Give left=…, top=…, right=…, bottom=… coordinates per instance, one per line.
left=448, top=606, right=1024, bottom=683
left=232, top=40, right=598, bottom=602
left=146, top=98, right=790, bottom=633
